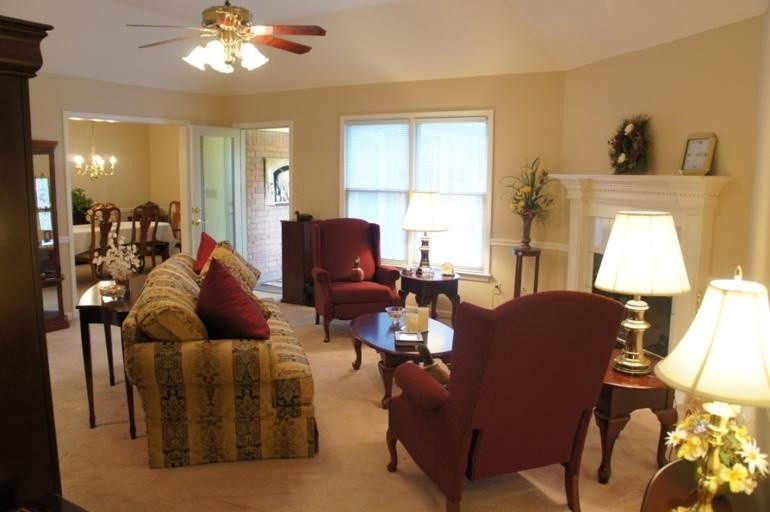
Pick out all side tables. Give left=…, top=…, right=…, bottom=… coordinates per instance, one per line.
left=75, top=275, right=144, bottom=440
left=399, top=270, right=461, bottom=323
left=596, top=359, right=679, bottom=484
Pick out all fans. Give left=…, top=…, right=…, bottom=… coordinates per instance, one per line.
left=125, top=1, right=327, bottom=55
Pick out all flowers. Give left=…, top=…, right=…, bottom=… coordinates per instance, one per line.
left=498, top=155, right=555, bottom=226
left=608, top=114, right=652, bottom=174
left=91, top=222, right=140, bottom=281
left=669, top=414, right=767, bottom=496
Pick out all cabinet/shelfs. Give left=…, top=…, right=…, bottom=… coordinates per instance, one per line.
left=32, top=138, right=70, bottom=332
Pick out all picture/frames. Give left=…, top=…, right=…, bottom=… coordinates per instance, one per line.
left=263, top=157, right=289, bottom=204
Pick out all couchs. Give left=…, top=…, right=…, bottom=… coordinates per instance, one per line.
left=387, top=291, right=627, bottom=512
left=120, top=251, right=318, bottom=470
left=309, top=218, right=401, bottom=343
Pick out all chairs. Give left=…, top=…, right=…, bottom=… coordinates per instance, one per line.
left=75, top=202, right=121, bottom=284
left=168, top=200, right=181, bottom=250
left=132, top=201, right=159, bottom=274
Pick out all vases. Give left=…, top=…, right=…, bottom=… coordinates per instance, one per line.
left=688, top=465, right=729, bottom=496
left=516, top=210, right=542, bottom=249
left=114, top=280, right=130, bottom=302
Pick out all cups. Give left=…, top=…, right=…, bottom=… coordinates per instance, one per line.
left=385, top=306, right=406, bottom=327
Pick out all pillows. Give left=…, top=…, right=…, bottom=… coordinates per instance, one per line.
left=220, top=239, right=261, bottom=278
left=201, top=245, right=257, bottom=290
left=195, top=263, right=273, bottom=322
left=195, top=257, right=270, bottom=340
left=193, top=232, right=217, bottom=274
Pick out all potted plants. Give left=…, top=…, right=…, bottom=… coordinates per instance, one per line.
left=72, top=187, right=94, bottom=225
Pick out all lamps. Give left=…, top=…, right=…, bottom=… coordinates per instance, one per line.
left=652, top=265, right=769, bottom=511
left=594, top=212, right=690, bottom=375
left=401, top=194, right=449, bottom=272
left=180, top=35, right=268, bottom=75
left=72, top=121, right=117, bottom=183
